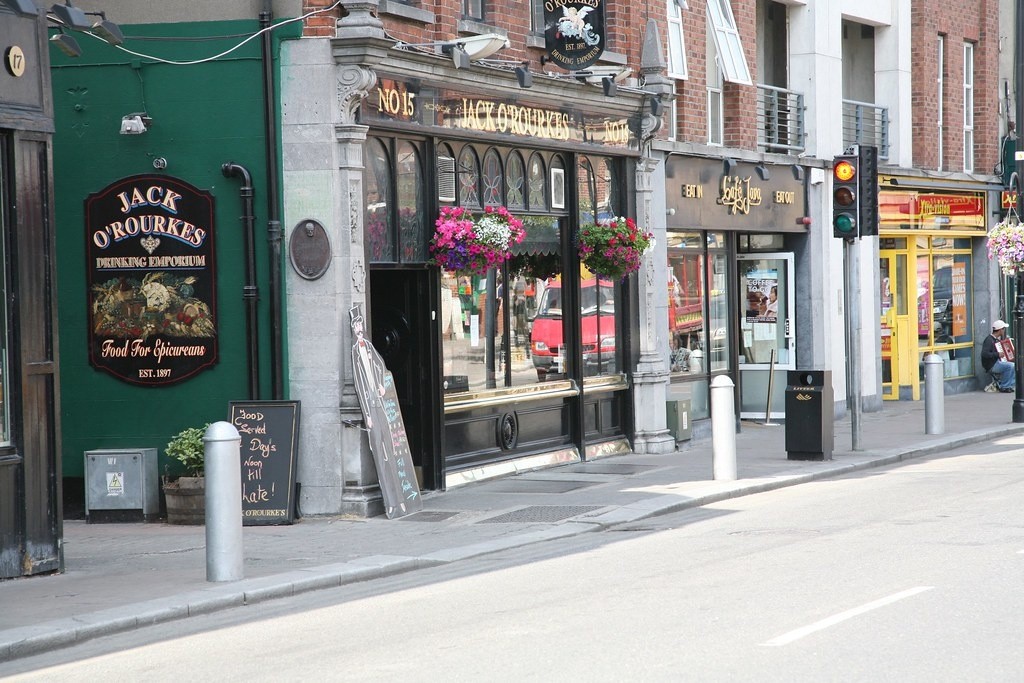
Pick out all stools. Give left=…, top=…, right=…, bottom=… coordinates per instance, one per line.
left=986, top=371, right=1002, bottom=390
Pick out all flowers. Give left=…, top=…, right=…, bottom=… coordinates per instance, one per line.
left=574, top=216, right=654, bottom=282
left=366, top=207, right=417, bottom=264
left=429, top=204, right=526, bottom=277
left=987, top=222, right=1024, bottom=279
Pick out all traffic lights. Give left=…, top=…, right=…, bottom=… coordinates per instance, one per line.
left=833, top=156, right=860, bottom=238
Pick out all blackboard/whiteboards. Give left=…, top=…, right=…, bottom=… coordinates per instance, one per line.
left=228, top=399, right=303, bottom=524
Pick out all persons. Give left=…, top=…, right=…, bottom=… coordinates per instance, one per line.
left=981, top=320, right=1015, bottom=393
left=765, top=286, right=778, bottom=317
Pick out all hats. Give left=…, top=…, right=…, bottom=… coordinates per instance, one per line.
left=934, top=322, right=942, bottom=331
left=993, top=320, right=1009, bottom=330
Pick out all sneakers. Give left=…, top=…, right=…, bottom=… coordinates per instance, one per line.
left=1000, top=387, right=1015, bottom=393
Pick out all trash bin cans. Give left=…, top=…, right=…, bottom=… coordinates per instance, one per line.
left=785, top=369, right=835, bottom=461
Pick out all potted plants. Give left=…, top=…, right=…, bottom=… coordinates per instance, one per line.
left=161, top=423, right=211, bottom=525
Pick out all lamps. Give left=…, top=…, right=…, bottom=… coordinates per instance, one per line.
left=0, top=0, right=123, bottom=56
left=683, top=153, right=738, bottom=183
left=732, top=159, right=770, bottom=181
left=121, top=113, right=153, bottom=135
left=618, top=87, right=664, bottom=118
left=396, top=40, right=470, bottom=71
left=765, top=162, right=804, bottom=182
left=470, top=58, right=533, bottom=89
left=882, top=177, right=899, bottom=189
left=543, top=73, right=618, bottom=97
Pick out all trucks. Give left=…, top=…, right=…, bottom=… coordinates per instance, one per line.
left=531, top=273, right=701, bottom=383
